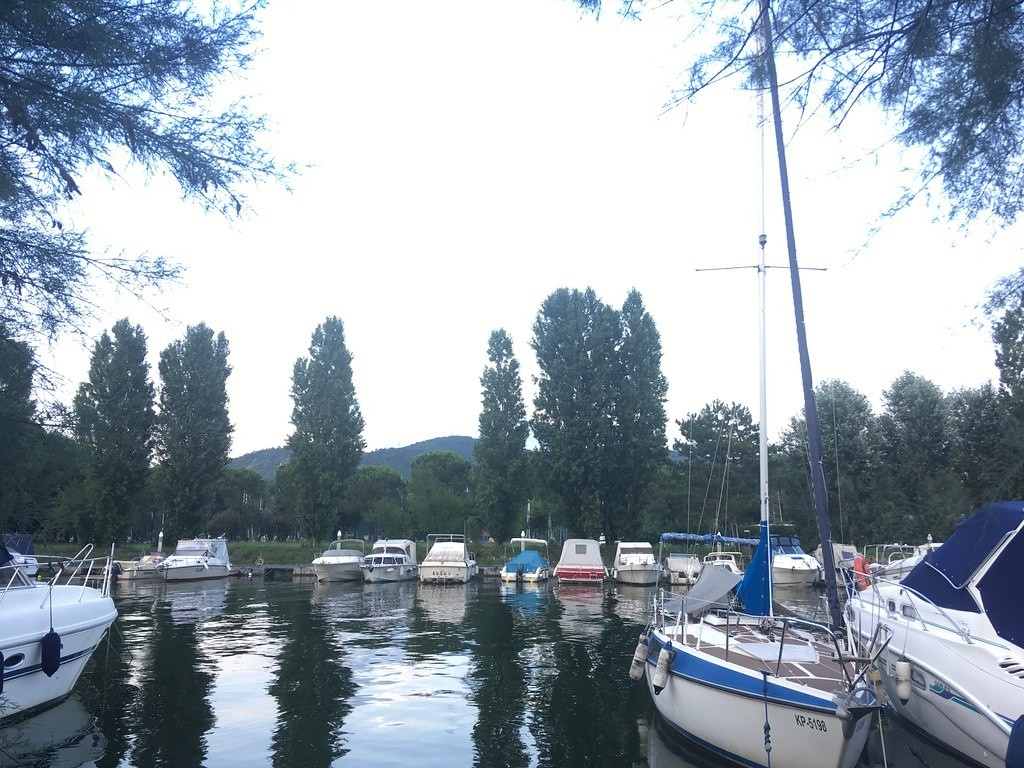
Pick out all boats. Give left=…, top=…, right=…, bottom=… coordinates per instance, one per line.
left=357, top=537, right=418, bottom=583
left=499, top=537, right=550, bottom=583
left=662, top=522, right=859, bottom=586
left=842, top=500, right=1024, bottom=768
left=0, top=533, right=232, bottom=727
left=552, top=539, right=609, bottom=584
left=418, top=533, right=480, bottom=584
left=311, top=539, right=365, bottom=583
left=610, top=541, right=662, bottom=585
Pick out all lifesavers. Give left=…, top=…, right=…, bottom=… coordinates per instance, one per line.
left=854, top=553, right=872, bottom=590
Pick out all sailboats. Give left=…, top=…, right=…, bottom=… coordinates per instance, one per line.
left=629, top=0, right=894, bottom=768
